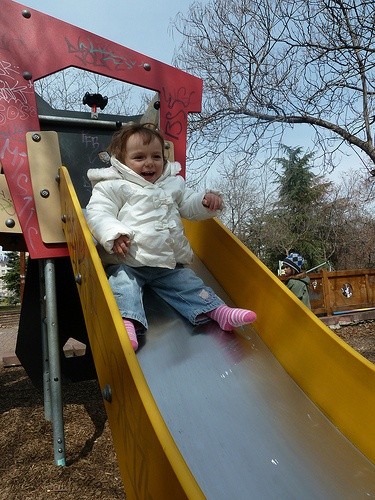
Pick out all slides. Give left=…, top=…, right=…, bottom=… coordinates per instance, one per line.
left=59, top=159, right=375, bottom=500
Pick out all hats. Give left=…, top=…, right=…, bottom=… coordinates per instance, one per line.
left=283, top=252, right=305, bottom=274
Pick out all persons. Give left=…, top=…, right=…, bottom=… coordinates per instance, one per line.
left=86, top=122, right=257, bottom=354
left=277, top=250, right=311, bottom=312
